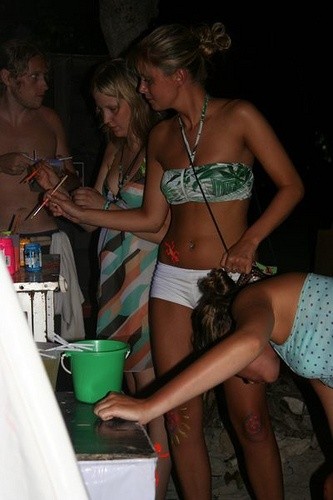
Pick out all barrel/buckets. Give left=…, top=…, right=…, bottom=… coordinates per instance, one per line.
left=61, top=340, right=131, bottom=405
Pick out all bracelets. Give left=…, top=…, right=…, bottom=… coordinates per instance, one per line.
left=104, top=200, right=112, bottom=210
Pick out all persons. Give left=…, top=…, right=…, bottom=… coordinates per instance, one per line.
left=44, top=19, right=306, bottom=500
left=95, top=266, right=333, bottom=500
left=33, top=60, right=172, bottom=500
left=0, top=34, right=76, bottom=255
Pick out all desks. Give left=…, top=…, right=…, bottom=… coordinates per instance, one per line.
left=11, top=252, right=63, bottom=342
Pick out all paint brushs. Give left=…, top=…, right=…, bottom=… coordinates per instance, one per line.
left=22, top=166, right=42, bottom=184
left=14, top=214, right=22, bottom=235
left=29, top=174, right=69, bottom=222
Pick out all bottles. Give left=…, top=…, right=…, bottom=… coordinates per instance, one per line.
left=0, top=230, right=43, bottom=274
